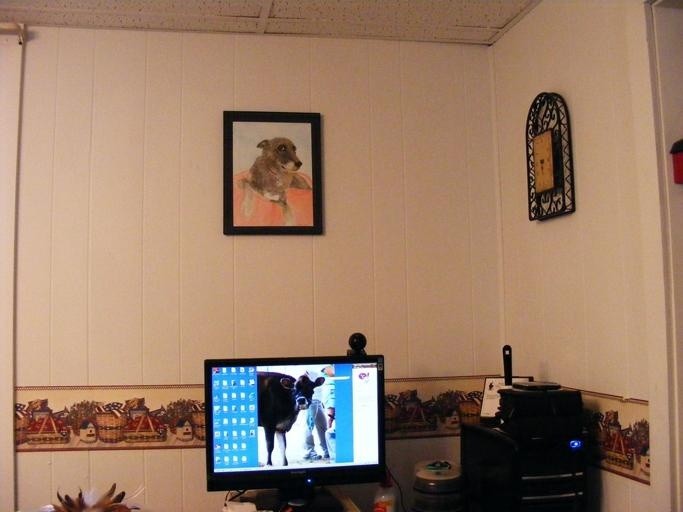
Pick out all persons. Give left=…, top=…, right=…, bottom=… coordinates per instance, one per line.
left=303, top=366, right=335, bottom=460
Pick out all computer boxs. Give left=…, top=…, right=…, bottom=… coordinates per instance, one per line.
left=460, top=420, right=601, bottom=511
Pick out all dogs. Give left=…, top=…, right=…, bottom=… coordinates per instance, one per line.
left=243, top=137, right=310, bottom=225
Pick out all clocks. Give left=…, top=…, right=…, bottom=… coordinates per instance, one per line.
left=524, top=91, right=574, bottom=221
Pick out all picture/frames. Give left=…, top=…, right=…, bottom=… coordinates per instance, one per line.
left=222, top=111, right=323, bottom=236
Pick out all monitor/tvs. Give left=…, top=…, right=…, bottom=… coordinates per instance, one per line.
left=203, top=354, right=385, bottom=512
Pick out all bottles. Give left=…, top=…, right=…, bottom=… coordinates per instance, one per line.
left=408, top=458, right=463, bottom=511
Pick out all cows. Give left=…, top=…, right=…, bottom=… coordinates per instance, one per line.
left=256, top=371, right=325, bottom=466
